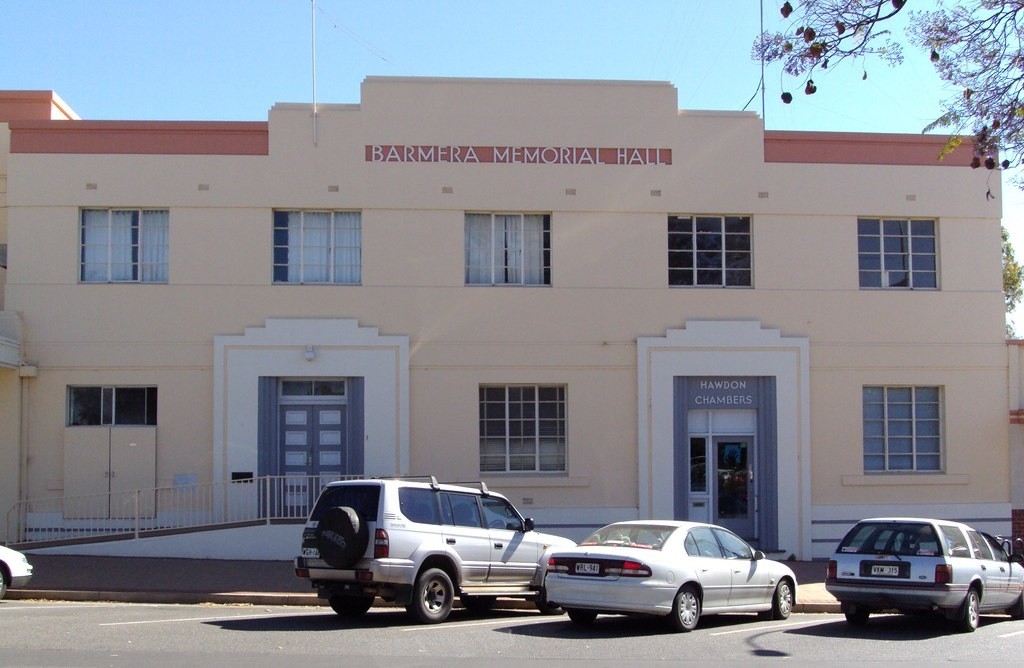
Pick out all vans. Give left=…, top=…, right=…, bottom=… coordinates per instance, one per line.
left=825, top=516, right=1024, bottom=634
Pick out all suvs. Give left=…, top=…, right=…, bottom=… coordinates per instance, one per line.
left=296, top=475, right=579, bottom=626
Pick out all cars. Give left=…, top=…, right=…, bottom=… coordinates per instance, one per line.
left=543, top=519, right=797, bottom=634
left=0, top=543, right=33, bottom=603
